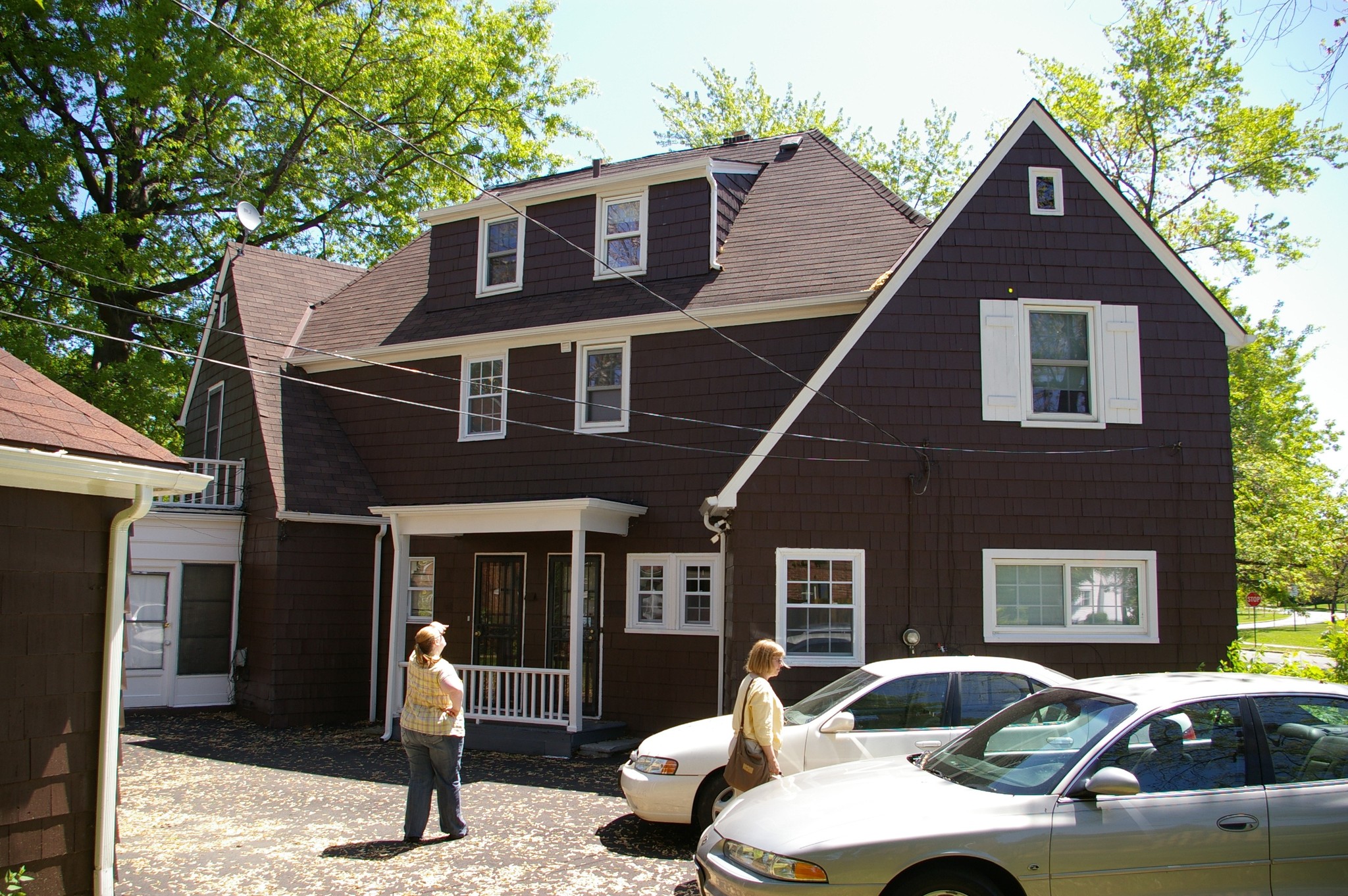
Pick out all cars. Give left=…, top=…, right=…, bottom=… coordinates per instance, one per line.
left=691, top=670, right=1348, bottom=896
left=786, top=623, right=853, bottom=656
left=620, top=655, right=1198, bottom=841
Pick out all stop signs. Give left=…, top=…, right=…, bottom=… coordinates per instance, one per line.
left=1246, top=592, right=1261, bottom=607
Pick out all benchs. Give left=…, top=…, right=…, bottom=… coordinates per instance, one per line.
left=1274, top=723, right=1348, bottom=780
left=1030, top=705, right=1061, bottom=723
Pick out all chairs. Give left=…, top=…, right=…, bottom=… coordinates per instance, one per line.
left=1196, top=723, right=1245, bottom=789
left=1128, top=719, right=1194, bottom=789
left=915, top=692, right=942, bottom=727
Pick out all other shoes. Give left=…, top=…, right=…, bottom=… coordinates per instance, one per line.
left=403, top=835, right=420, bottom=842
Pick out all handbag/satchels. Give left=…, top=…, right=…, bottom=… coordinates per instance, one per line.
left=724, top=728, right=772, bottom=792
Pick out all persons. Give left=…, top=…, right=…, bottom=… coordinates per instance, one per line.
left=728, top=639, right=792, bottom=797
left=400, top=622, right=469, bottom=846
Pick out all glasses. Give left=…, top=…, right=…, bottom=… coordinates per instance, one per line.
left=772, top=658, right=782, bottom=664
